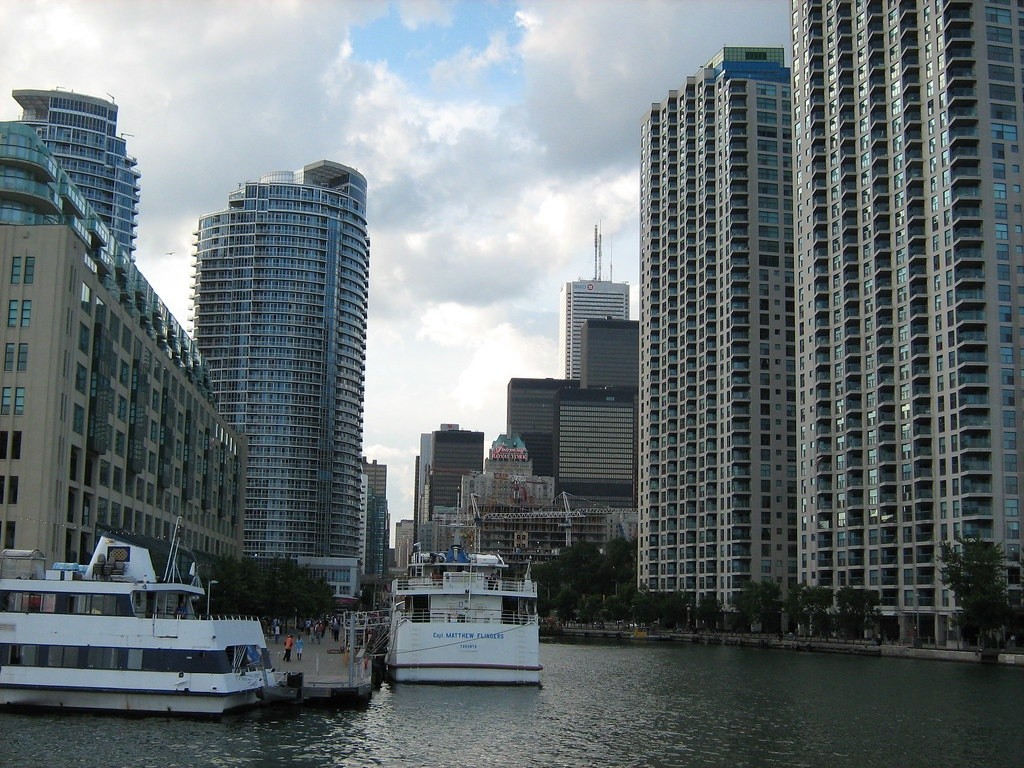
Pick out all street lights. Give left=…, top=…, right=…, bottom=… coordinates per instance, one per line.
left=916, top=594, right=921, bottom=640
left=206, top=579, right=218, bottom=619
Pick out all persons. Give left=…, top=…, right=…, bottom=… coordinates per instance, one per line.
left=152, top=603, right=162, bottom=619
left=304, top=612, right=343, bottom=644
left=343, top=615, right=373, bottom=679
left=273, top=616, right=304, bottom=662
left=544, top=613, right=883, bottom=649
left=174, top=603, right=186, bottom=619
left=430, top=567, right=500, bottom=590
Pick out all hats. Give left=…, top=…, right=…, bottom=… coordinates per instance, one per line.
left=318, top=621, right=322, bottom=624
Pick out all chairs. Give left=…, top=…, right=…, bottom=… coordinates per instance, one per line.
left=92, top=554, right=126, bottom=582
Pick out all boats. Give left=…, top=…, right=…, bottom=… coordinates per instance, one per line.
left=0, top=537, right=277, bottom=716
left=383, top=542, right=546, bottom=684
